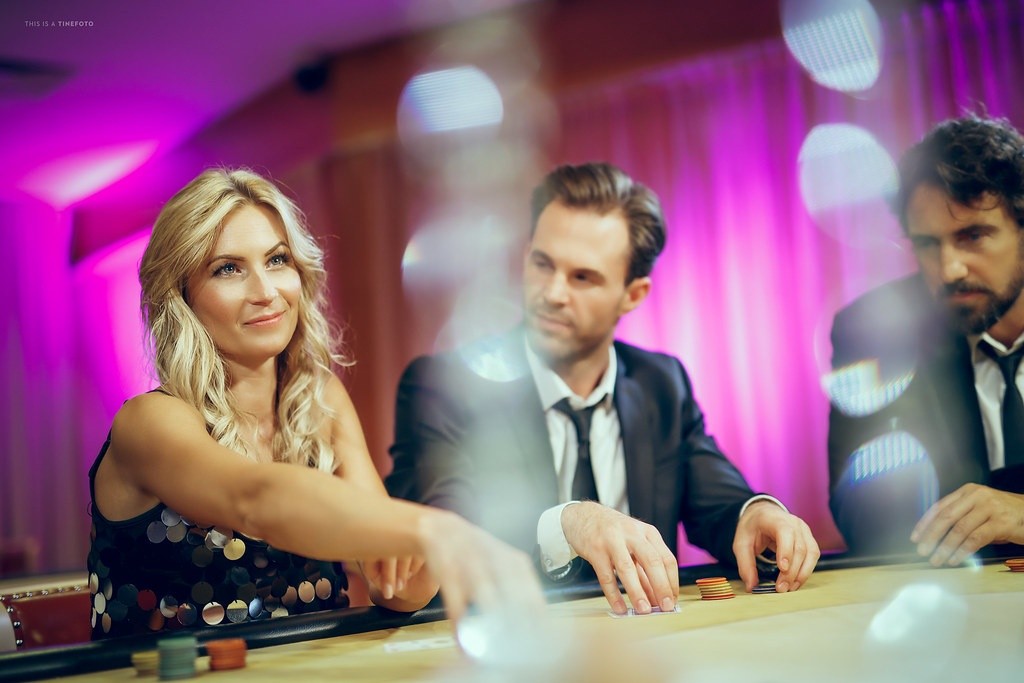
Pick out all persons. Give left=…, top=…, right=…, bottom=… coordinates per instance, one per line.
left=83, top=167, right=540, bottom=653
left=828, top=109, right=1024, bottom=569
left=379, top=160, right=821, bottom=615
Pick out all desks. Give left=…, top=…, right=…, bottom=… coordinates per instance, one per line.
left=0, top=551, right=1024, bottom=683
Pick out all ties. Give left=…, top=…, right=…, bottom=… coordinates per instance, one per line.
left=551, top=394, right=609, bottom=582
left=977, top=340, right=1024, bottom=468
